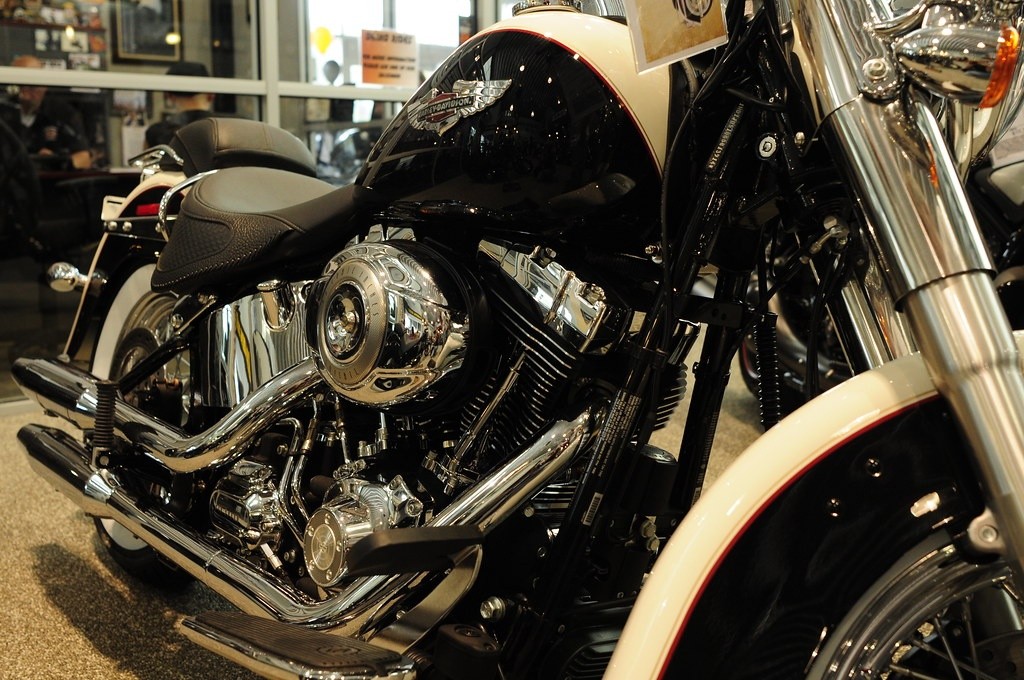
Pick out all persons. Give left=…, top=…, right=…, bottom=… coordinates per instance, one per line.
left=143, top=61, right=247, bottom=158
left=1, top=54, right=94, bottom=172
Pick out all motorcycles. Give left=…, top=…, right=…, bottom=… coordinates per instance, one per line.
left=14, top=0, right=1024, bottom=680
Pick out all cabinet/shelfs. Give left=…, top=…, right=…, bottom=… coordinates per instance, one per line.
left=1, top=19, right=114, bottom=181
